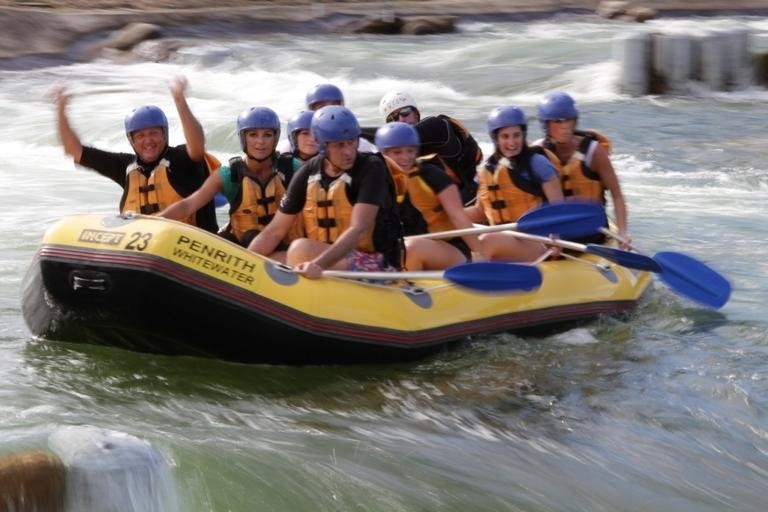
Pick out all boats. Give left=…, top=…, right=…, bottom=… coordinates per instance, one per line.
left=22, top=203, right=654, bottom=357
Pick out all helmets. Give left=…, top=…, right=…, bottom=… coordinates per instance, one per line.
left=487, top=106, right=527, bottom=133
left=125, top=106, right=168, bottom=133
left=537, top=94, right=577, bottom=122
left=237, top=84, right=421, bottom=156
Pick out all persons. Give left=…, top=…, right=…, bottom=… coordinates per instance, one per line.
left=156, top=108, right=309, bottom=248
left=532, top=91, right=629, bottom=250
left=464, top=104, right=562, bottom=265
left=375, top=122, right=485, bottom=273
left=246, top=104, right=406, bottom=275
left=284, top=80, right=482, bottom=206
left=51, top=74, right=216, bottom=235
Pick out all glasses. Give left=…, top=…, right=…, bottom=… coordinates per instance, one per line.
left=387, top=107, right=412, bottom=121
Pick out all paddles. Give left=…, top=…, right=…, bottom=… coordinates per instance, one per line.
left=403, top=200, right=608, bottom=238
left=597, top=226, right=732, bottom=309
left=471, top=221, right=662, bottom=274
left=321, top=261, right=542, bottom=290
